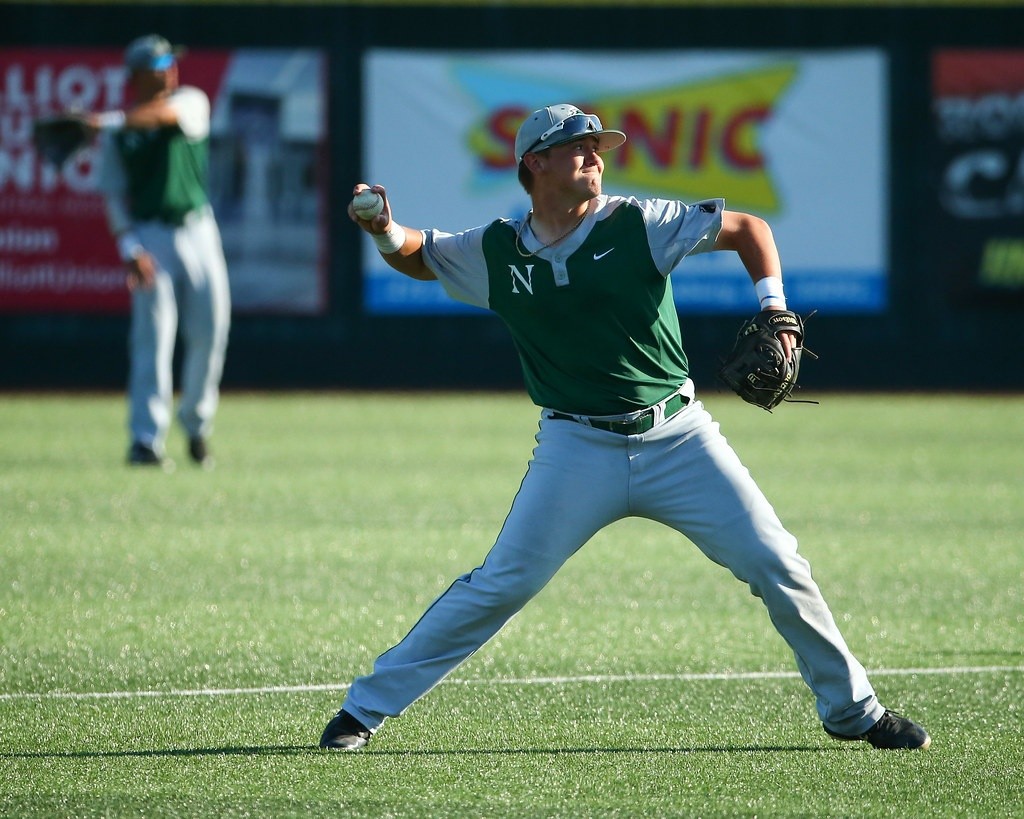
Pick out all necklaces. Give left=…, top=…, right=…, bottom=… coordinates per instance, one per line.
left=516, top=199, right=591, bottom=257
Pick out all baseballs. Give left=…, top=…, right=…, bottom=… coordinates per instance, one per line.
left=353, top=188, right=384, bottom=222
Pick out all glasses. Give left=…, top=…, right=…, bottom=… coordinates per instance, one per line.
left=520, top=114, right=603, bottom=161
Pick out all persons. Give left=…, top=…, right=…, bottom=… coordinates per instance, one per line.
left=319, top=103, right=933, bottom=751
left=69, top=34, right=232, bottom=469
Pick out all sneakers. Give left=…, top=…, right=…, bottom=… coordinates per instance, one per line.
left=320, top=707, right=369, bottom=751
left=823, top=708, right=931, bottom=751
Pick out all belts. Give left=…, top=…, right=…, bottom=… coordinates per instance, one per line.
left=551, top=394, right=691, bottom=436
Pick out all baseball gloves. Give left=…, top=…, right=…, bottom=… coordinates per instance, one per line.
left=717, top=310, right=806, bottom=413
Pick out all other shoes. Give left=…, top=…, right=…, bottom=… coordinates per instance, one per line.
left=190, top=437, right=211, bottom=466
left=132, top=447, right=166, bottom=470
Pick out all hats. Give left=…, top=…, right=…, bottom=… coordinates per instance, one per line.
left=514, top=104, right=628, bottom=162
left=126, top=36, right=174, bottom=72
